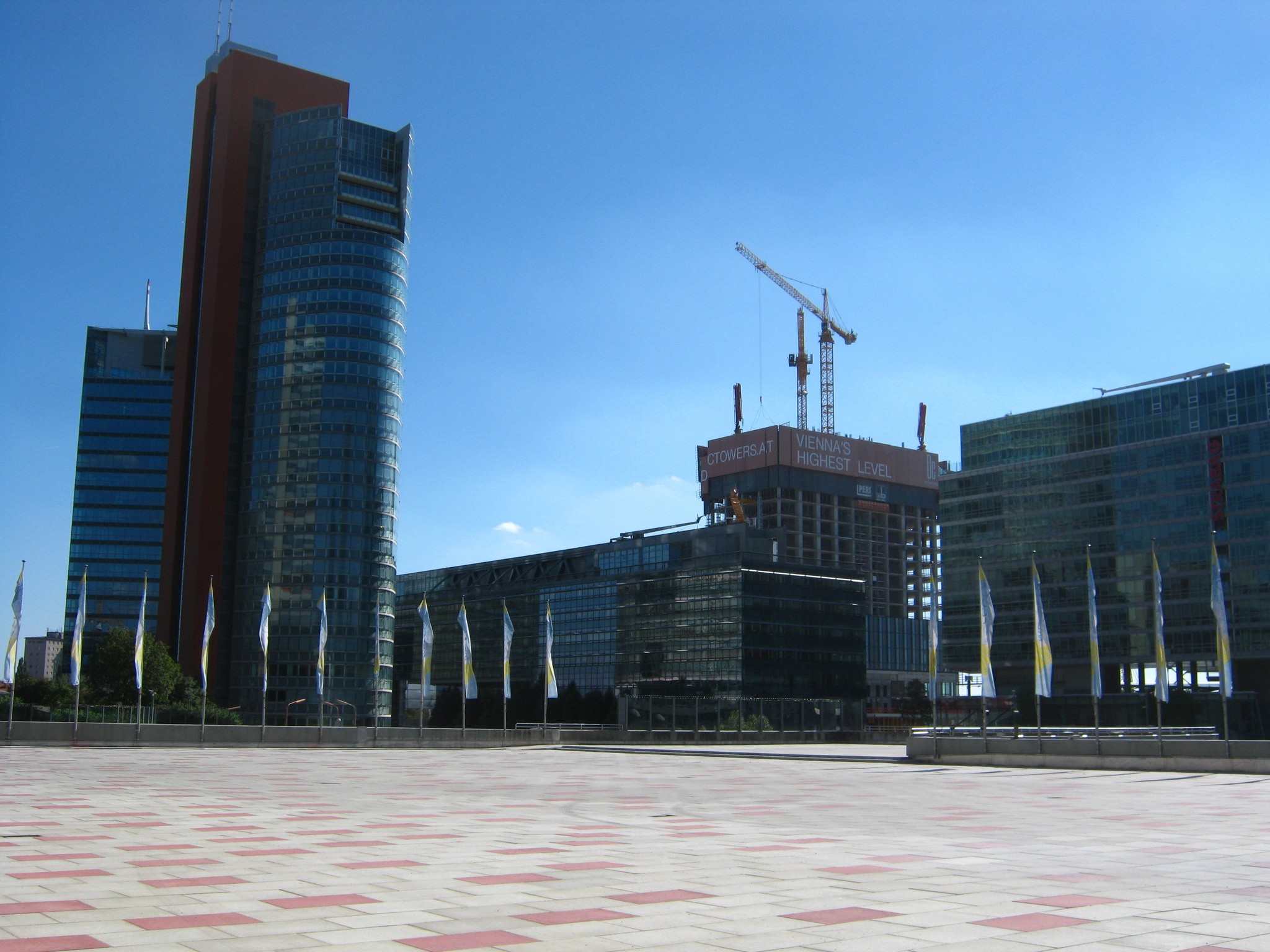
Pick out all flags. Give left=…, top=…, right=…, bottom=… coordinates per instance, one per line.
left=457, top=604, right=477, bottom=698
left=930, top=575, right=938, bottom=700
left=70, top=572, right=86, bottom=685
left=1033, top=562, right=1052, bottom=697
left=1153, top=553, right=1168, bottom=702
left=504, top=605, right=514, bottom=698
left=316, top=593, right=328, bottom=695
left=375, top=605, right=379, bottom=678
left=417, top=598, right=434, bottom=696
left=1087, top=557, right=1101, bottom=699
left=1211, top=544, right=1233, bottom=696
left=134, top=577, right=147, bottom=689
left=546, top=608, right=557, bottom=698
left=3, top=571, right=24, bottom=682
left=201, top=583, right=215, bottom=690
left=978, top=566, right=996, bottom=698
left=259, top=582, right=271, bottom=691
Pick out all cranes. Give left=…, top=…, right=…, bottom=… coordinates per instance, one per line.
left=732, top=238, right=859, bottom=434
